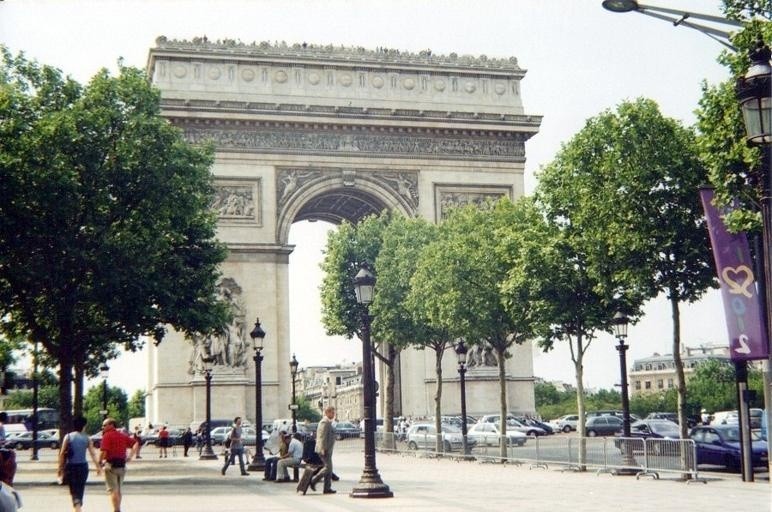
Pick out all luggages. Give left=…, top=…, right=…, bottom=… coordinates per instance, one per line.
left=296, top=453, right=329, bottom=495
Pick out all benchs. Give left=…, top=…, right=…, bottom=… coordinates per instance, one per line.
left=286, top=463, right=323, bottom=482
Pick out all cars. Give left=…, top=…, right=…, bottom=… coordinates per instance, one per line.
left=687, top=424, right=768, bottom=473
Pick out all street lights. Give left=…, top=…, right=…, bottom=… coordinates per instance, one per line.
left=734, top=33, right=771, bottom=373
left=246, top=317, right=272, bottom=471
left=455, top=337, right=475, bottom=460
left=609, top=301, right=643, bottom=476
left=601, top=0, right=771, bottom=57
left=289, top=352, right=299, bottom=438
left=350, top=261, right=393, bottom=498
left=199, top=345, right=219, bottom=460
left=100, top=361, right=110, bottom=435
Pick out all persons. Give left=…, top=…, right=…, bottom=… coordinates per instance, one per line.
left=196, top=427, right=204, bottom=457
left=401, top=417, right=410, bottom=444
left=699, top=408, right=711, bottom=425
left=57, top=416, right=102, bottom=511
left=97, top=418, right=138, bottom=512
left=263, top=418, right=341, bottom=483
left=134, top=425, right=144, bottom=459
left=280, top=168, right=313, bottom=205
left=1, top=447, right=22, bottom=511
left=1, top=411, right=10, bottom=450
left=120, top=421, right=130, bottom=460
left=175, top=128, right=526, bottom=158
left=216, top=186, right=254, bottom=217
left=182, top=427, right=194, bottom=457
left=441, top=192, right=505, bottom=216
left=464, top=336, right=496, bottom=369
left=224, top=426, right=255, bottom=464
left=221, top=417, right=250, bottom=476
left=396, top=415, right=404, bottom=442
left=188, top=292, right=250, bottom=371
left=309, top=405, right=339, bottom=494
left=382, top=173, right=416, bottom=207
left=159, top=426, right=170, bottom=458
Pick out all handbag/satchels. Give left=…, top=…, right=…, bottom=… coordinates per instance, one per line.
left=63, top=443, right=73, bottom=459
left=112, top=458, right=126, bottom=469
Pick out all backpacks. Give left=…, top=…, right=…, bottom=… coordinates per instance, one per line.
left=224, top=427, right=236, bottom=449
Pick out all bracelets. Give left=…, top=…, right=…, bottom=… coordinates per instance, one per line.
left=98, top=460, right=105, bottom=467
